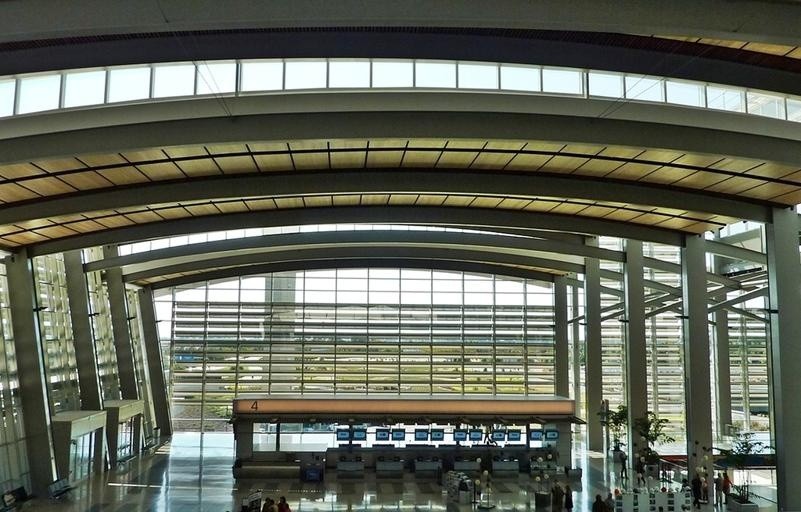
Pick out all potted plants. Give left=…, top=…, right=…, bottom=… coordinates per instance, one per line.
left=597, top=405, right=776, bottom=512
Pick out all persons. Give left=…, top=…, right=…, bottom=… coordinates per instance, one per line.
left=635, top=457, right=646, bottom=485
left=260, top=495, right=292, bottom=512
left=690, top=472, right=733, bottom=510
left=604, top=493, right=615, bottom=512
left=549, top=479, right=573, bottom=512
left=591, top=494, right=606, bottom=512
left=618, top=453, right=628, bottom=479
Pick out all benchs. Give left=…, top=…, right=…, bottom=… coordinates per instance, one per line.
left=47, top=477, right=77, bottom=503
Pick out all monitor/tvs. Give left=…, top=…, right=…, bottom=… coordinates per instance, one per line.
left=508, top=429, right=521, bottom=440
left=415, top=429, right=428, bottom=440
left=529, top=429, right=544, bottom=440
left=376, top=429, right=389, bottom=440
left=337, top=429, right=350, bottom=440
left=392, top=429, right=405, bottom=440
left=352, top=429, right=366, bottom=440
left=545, top=429, right=559, bottom=440
left=492, top=429, right=506, bottom=440
left=431, top=429, right=444, bottom=440
left=469, top=429, right=482, bottom=440
left=454, top=429, right=466, bottom=440
